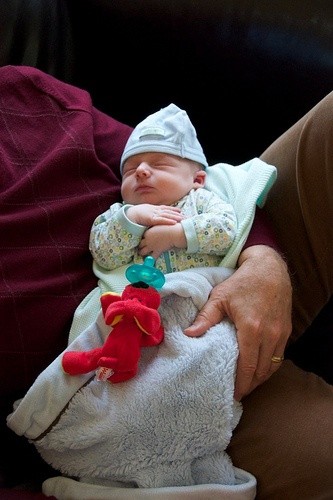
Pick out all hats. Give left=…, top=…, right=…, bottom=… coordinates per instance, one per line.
left=120, top=103, right=209, bottom=174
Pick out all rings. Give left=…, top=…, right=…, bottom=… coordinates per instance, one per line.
left=272, top=355, right=284, bottom=362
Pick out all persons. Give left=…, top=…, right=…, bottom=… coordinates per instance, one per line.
left=0, top=66, right=333, bottom=500
left=89, top=104, right=237, bottom=273
